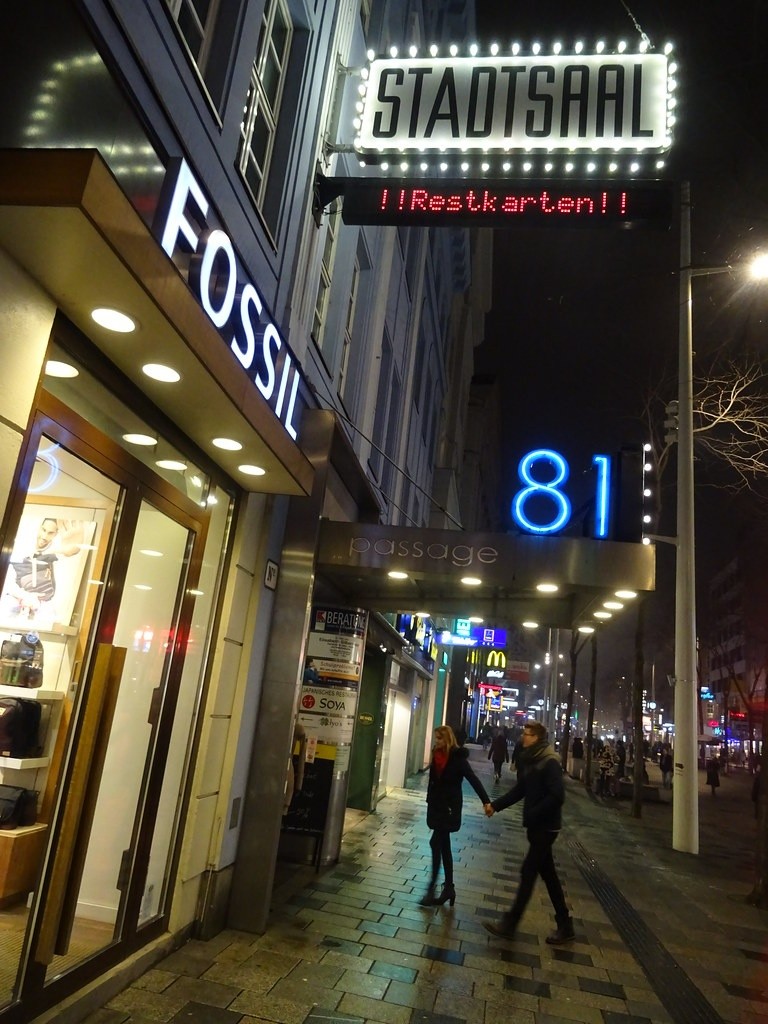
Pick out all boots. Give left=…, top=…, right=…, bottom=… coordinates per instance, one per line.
left=481, top=913, right=521, bottom=940
left=546, top=916, right=577, bottom=944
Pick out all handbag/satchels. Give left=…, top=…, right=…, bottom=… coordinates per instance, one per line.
left=510, top=763, right=516, bottom=771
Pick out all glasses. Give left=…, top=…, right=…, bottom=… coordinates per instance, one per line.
left=521, top=732, right=537, bottom=737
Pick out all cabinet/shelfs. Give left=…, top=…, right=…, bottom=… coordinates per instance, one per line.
left=0, top=626, right=80, bottom=909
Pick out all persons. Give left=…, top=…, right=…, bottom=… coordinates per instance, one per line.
left=568, top=733, right=762, bottom=819
left=706, top=757, right=720, bottom=796
left=420, top=726, right=491, bottom=907
left=482, top=721, right=576, bottom=945
left=476, top=722, right=525, bottom=783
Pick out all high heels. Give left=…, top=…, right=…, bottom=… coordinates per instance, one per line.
left=418, top=883, right=439, bottom=906
left=433, top=883, right=456, bottom=906
